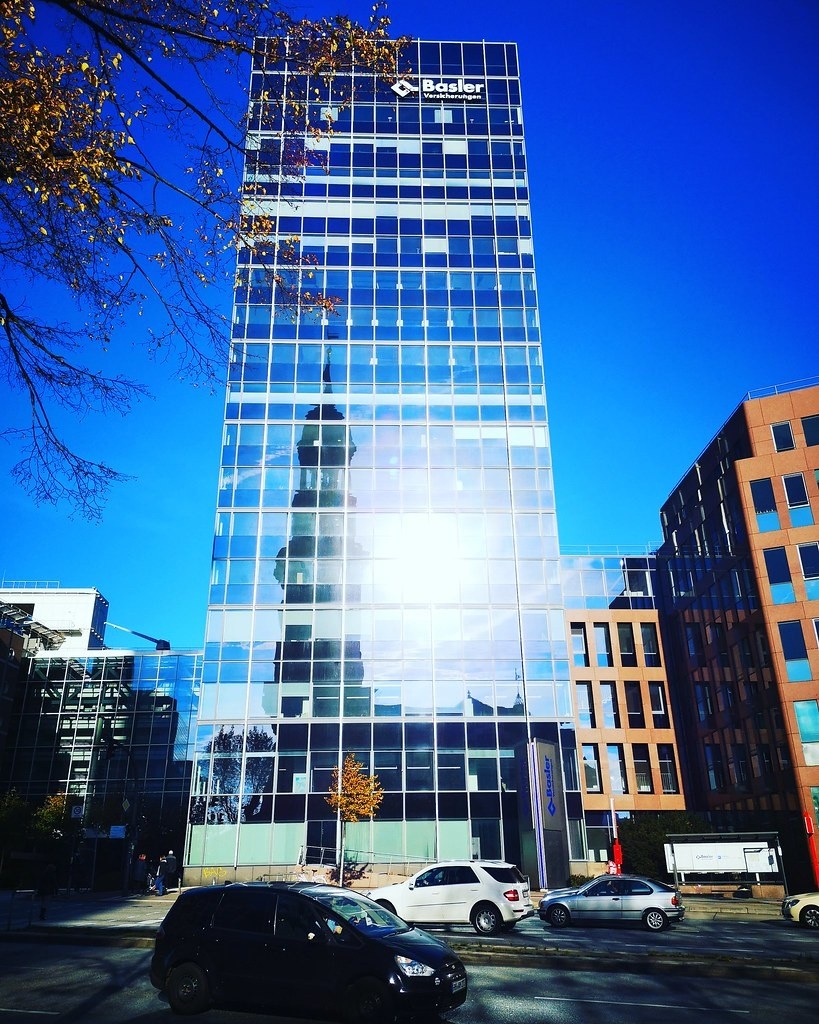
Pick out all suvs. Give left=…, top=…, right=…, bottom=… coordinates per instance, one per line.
left=366, top=860, right=535, bottom=938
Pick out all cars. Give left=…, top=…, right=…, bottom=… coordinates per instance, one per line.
left=148, top=879, right=470, bottom=1023
left=538, top=874, right=685, bottom=930
left=781, top=892, right=819, bottom=928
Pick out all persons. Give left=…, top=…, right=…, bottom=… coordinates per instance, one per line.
left=130, top=850, right=179, bottom=895
